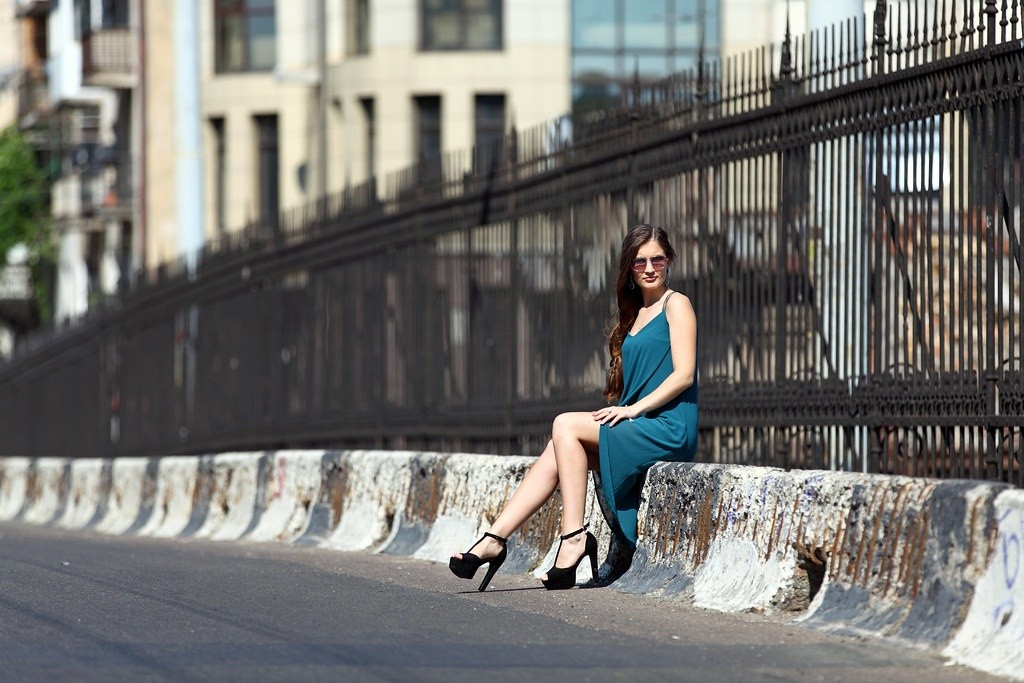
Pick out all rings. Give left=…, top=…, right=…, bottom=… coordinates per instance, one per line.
left=608, top=410, right=611, bottom=413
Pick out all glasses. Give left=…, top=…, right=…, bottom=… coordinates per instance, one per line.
left=630, top=255, right=669, bottom=272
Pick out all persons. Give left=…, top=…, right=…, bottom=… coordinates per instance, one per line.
left=450, top=224, right=701, bottom=594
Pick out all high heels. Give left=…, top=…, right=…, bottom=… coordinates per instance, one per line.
left=449, top=532, right=507, bottom=592
left=541, top=522, right=600, bottom=590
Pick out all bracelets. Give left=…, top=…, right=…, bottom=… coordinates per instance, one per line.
left=624, top=405, right=631, bottom=419
